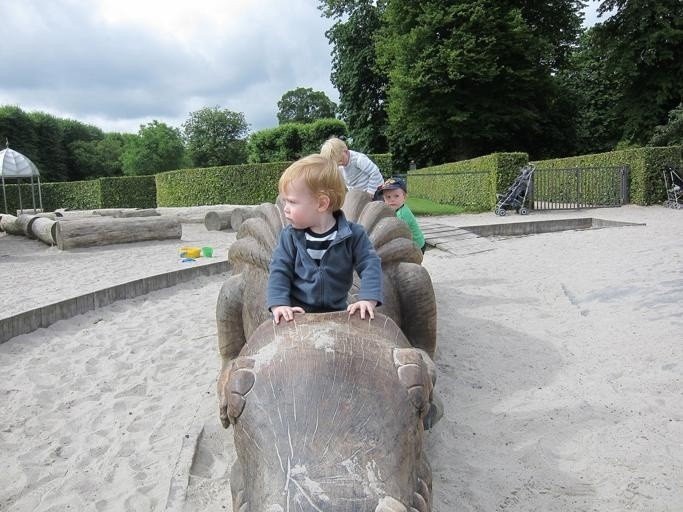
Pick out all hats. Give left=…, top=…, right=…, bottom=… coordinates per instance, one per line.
left=377, top=178, right=407, bottom=195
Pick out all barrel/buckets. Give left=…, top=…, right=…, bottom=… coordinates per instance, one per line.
left=179, top=247, right=213, bottom=258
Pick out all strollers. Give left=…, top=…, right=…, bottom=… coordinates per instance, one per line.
left=495, top=164, right=536, bottom=216
left=660, top=164, right=683, bottom=210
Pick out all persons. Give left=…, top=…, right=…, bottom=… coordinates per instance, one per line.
left=266, top=155, right=385, bottom=325
left=319, top=137, right=384, bottom=203
left=380, top=178, right=426, bottom=257
